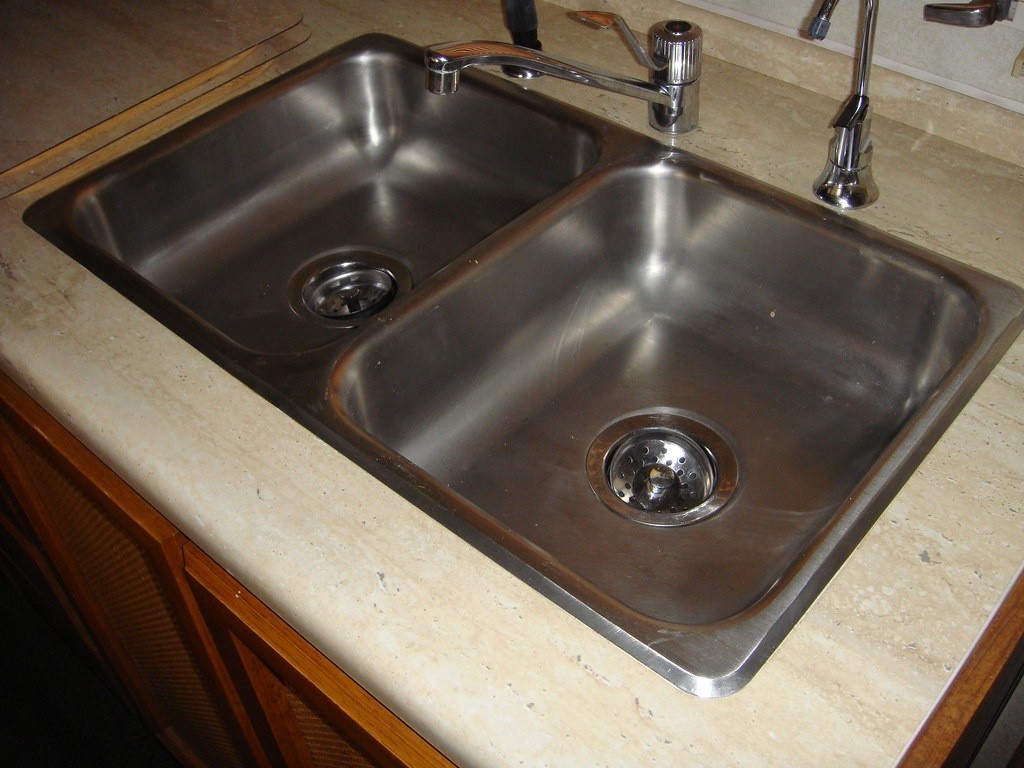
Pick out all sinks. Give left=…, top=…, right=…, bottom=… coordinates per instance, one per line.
left=322, top=142, right=1023, bottom=700
left=19, top=30, right=633, bottom=364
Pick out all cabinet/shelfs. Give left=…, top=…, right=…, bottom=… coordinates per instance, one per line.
left=0, top=370, right=458, bottom=768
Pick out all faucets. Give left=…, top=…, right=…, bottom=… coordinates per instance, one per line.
left=420, top=8, right=706, bottom=134
left=804, top=1, right=883, bottom=210
